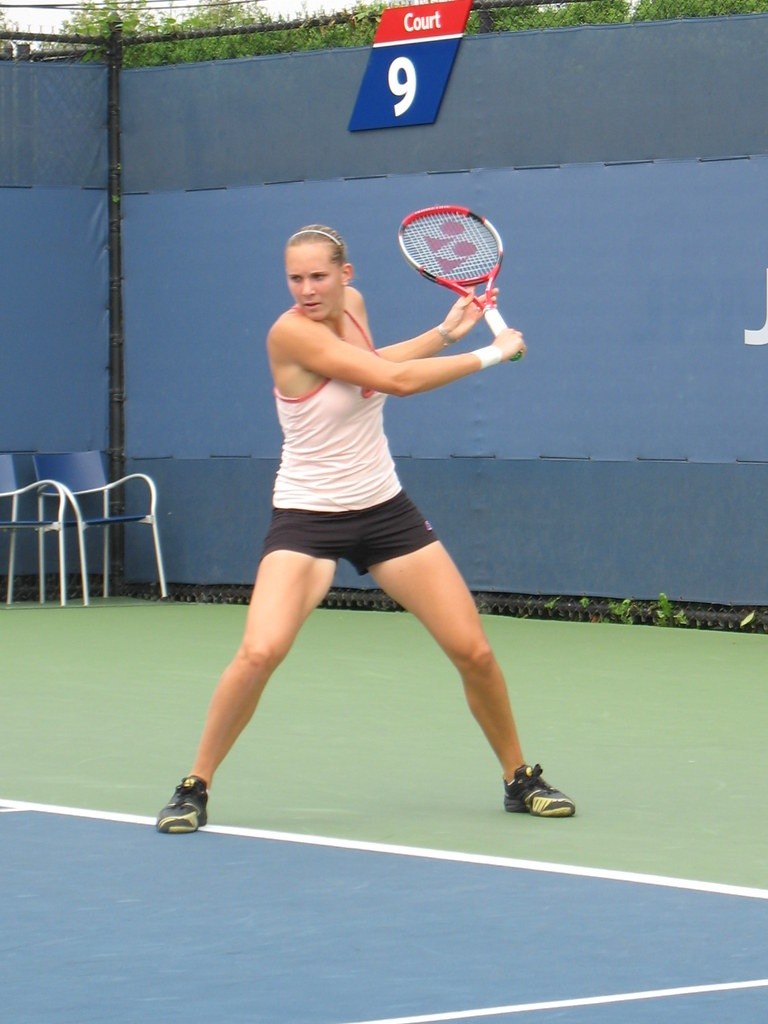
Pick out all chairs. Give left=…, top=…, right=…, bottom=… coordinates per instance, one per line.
left=0, top=451, right=67, bottom=607
left=32, top=450, right=169, bottom=606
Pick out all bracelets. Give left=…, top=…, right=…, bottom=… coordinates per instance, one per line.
left=437, top=325, right=455, bottom=344
left=471, top=345, right=502, bottom=370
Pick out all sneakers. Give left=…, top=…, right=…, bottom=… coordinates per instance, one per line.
left=157, top=775, right=209, bottom=833
left=504, top=763, right=575, bottom=816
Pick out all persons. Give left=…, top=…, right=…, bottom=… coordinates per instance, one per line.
left=157, top=224, right=576, bottom=833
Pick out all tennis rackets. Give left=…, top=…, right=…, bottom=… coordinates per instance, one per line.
left=398, top=207, right=523, bottom=364
